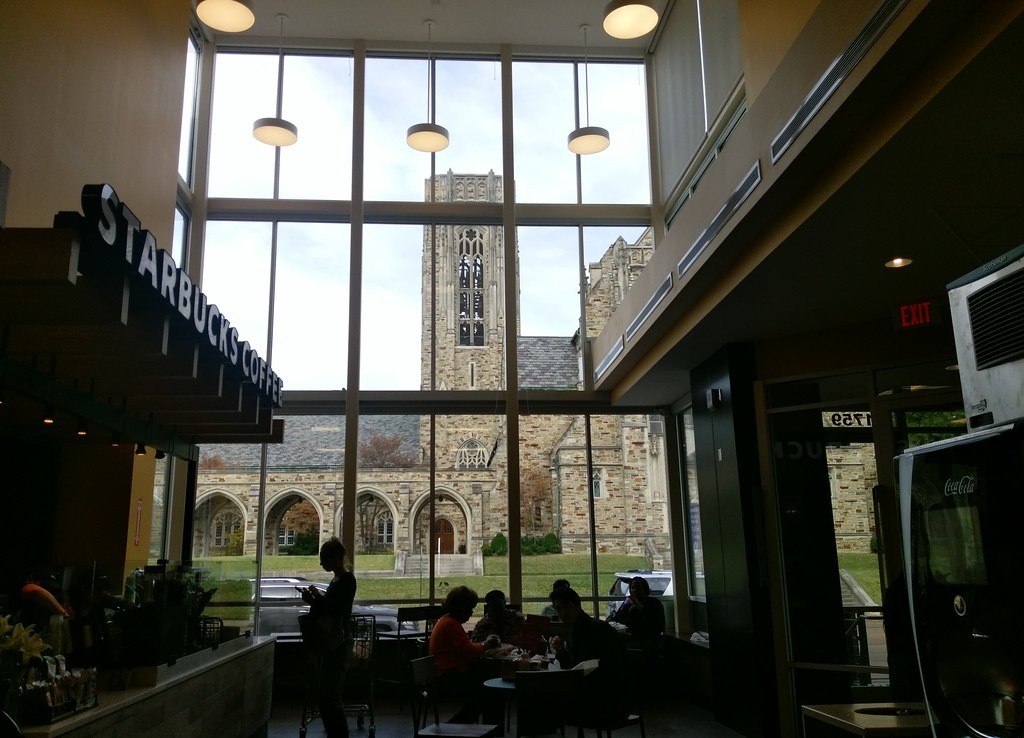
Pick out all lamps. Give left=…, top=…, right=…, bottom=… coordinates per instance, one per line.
left=155, top=450, right=165, bottom=459
left=43, top=407, right=55, bottom=423
left=135, top=444, right=146, bottom=455
left=111, top=433, right=120, bottom=447
left=78, top=420, right=88, bottom=435
left=602, top=0, right=659, bottom=39
left=568, top=24, right=610, bottom=154
left=196, top=0, right=255, bottom=33
left=407, top=20, right=449, bottom=152
left=253, top=14, right=297, bottom=146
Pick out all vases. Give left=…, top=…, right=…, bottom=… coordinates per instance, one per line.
left=0, top=679, right=23, bottom=730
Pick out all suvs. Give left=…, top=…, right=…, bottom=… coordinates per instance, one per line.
left=250, top=577, right=420, bottom=637
left=607, top=570, right=705, bottom=619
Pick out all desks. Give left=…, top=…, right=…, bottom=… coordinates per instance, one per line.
left=484, top=677, right=517, bottom=732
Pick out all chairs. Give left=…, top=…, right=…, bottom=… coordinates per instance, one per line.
left=583, top=646, right=648, bottom=738
left=406, top=655, right=499, bottom=738
left=376, top=606, right=444, bottom=713
left=514, top=669, right=585, bottom=738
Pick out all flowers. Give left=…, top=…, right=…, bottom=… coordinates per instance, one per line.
left=0, top=614, right=52, bottom=720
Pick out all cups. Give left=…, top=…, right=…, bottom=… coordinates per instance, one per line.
left=140, top=667, right=156, bottom=686
left=529, top=660, right=541, bottom=671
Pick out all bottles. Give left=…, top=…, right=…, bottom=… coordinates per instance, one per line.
left=519, top=654, right=528, bottom=671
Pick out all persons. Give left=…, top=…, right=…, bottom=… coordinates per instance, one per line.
left=302, top=538, right=358, bottom=738
left=429, top=577, right=665, bottom=723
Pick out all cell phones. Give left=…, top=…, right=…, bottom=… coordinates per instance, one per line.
left=294, top=587, right=304, bottom=593
